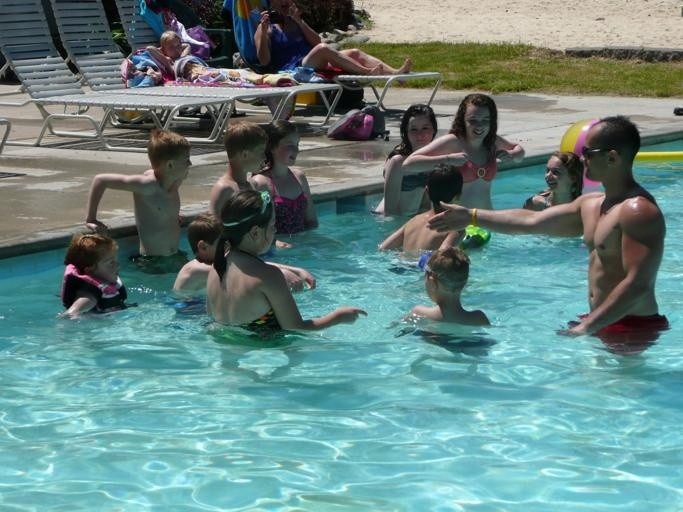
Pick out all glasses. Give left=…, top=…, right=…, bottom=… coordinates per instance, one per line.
left=581, top=146, right=622, bottom=156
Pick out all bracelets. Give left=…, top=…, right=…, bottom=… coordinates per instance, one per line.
left=470, top=208, right=479, bottom=226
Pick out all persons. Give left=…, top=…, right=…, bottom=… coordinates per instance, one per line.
left=403, top=247, right=493, bottom=326
left=249, top=122, right=319, bottom=235
left=146, top=30, right=266, bottom=85
left=85, top=129, right=193, bottom=259
left=209, top=122, right=293, bottom=253
left=523, top=151, right=584, bottom=212
left=254, top=1, right=412, bottom=75
left=373, top=103, right=437, bottom=220
left=378, top=162, right=466, bottom=268
left=61, top=233, right=127, bottom=317
left=402, top=93, right=525, bottom=210
left=427, top=115, right=667, bottom=335
left=207, top=188, right=369, bottom=332
left=171, top=216, right=315, bottom=291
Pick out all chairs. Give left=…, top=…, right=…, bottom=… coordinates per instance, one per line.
left=235, top=0, right=441, bottom=112
left=0, top=119, right=11, bottom=156
left=114, top=0, right=343, bottom=127
left=50, top=0, right=289, bottom=140
left=0, top=0, right=235, bottom=153
